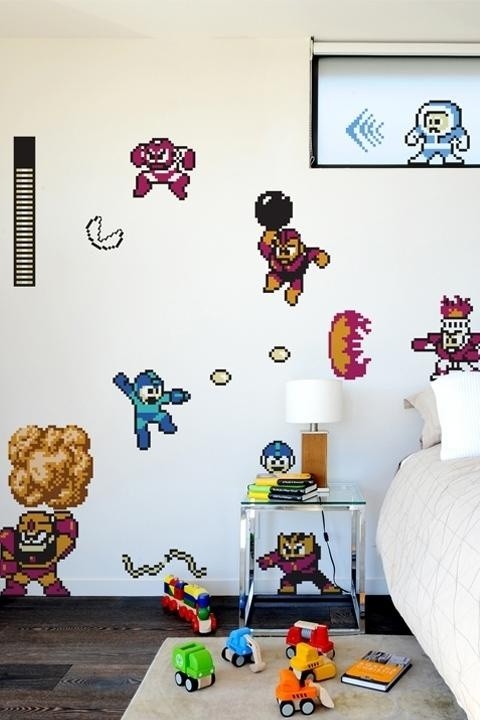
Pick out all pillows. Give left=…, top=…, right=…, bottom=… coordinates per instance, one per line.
left=402, top=370, right=479, bottom=458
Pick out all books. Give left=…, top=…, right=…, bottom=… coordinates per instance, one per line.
left=340, top=649, right=413, bottom=693
left=247, top=483, right=319, bottom=495
left=245, top=490, right=319, bottom=501
left=256, top=472, right=314, bottom=485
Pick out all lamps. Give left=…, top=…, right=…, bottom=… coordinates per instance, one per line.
left=284, top=377, right=345, bottom=488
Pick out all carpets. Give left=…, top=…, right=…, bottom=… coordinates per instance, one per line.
left=118, top=631, right=466, bottom=720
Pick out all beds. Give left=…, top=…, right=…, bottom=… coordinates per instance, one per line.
left=391, top=444, right=480, bottom=720
